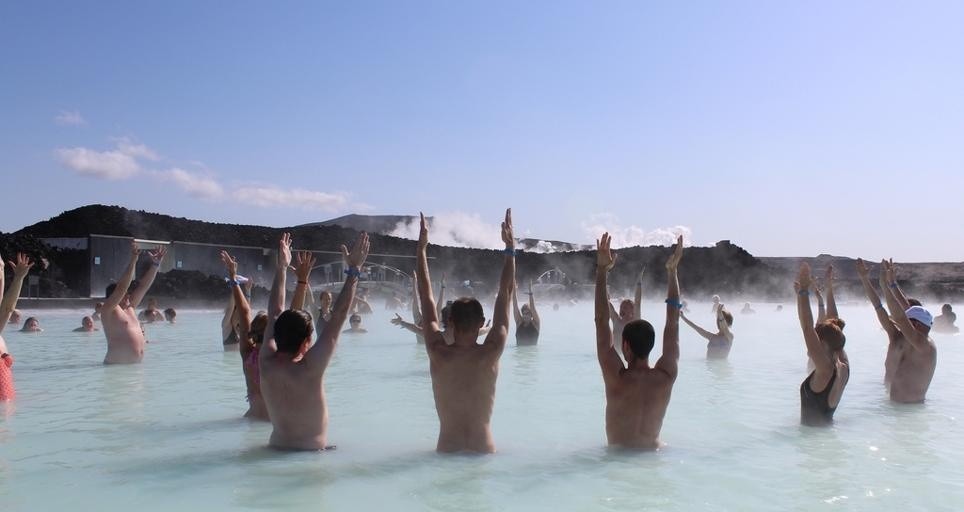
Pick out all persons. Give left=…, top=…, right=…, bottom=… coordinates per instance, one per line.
left=1, top=212, right=959, bottom=450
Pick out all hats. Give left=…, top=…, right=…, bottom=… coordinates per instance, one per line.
left=905, top=305, right=933, bottom=330
